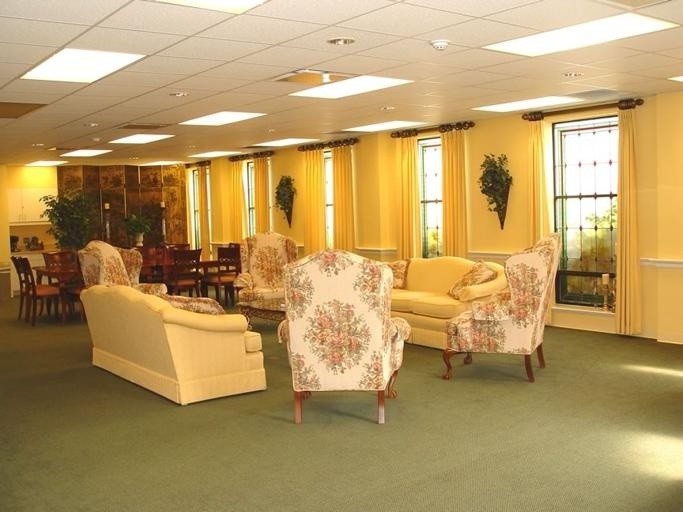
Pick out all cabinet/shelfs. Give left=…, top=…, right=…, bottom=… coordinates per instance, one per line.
left=7, top=188, right=58, bottom=226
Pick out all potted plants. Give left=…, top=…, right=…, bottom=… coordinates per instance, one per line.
left=476, top=152, right=513, bottom=230
left=121, top=213, right=152, bottom=247
left=273, top=175, right=297, bottom=228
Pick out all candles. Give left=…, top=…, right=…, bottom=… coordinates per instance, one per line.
left=160, top=202, right=165, bottom=208
left=104, top=203, right=109, bottom=210
left=602, top=273, right=610, bottom=286
